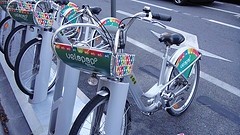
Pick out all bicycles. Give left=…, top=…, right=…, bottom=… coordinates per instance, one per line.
left=52, top=4, right=202, bottom=135
left=0, top=0, right=124, bottom=98
